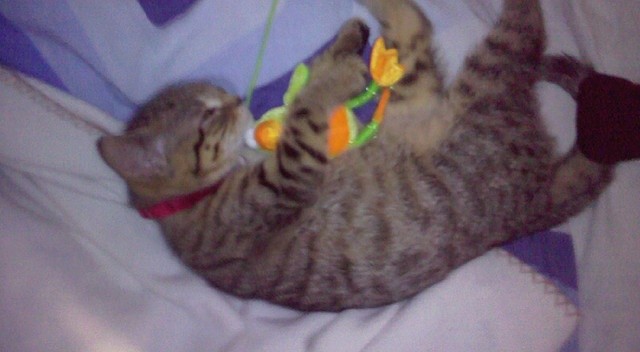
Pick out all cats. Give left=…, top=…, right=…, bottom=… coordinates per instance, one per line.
left=96, top=0, right=613, bottom=313
